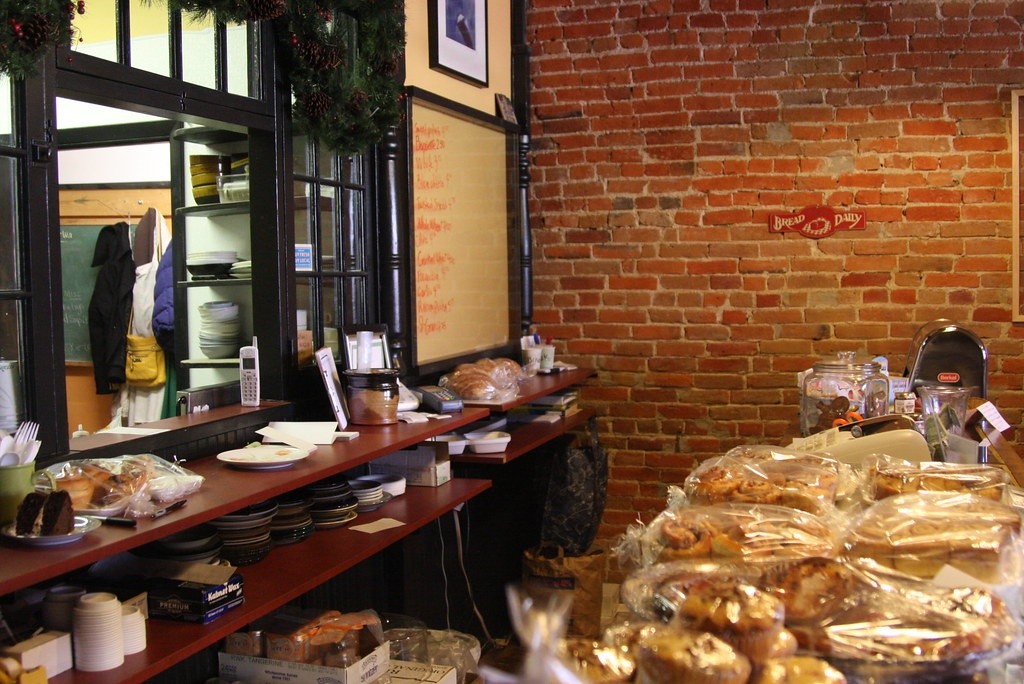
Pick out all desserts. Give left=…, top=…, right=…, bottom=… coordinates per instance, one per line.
left=14, top=489, right=75, bottom=537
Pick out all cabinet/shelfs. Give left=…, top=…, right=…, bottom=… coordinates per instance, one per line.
left=173, top=125, right=332, bottom=368
left=0, top=409, right=492, bottom=684
left=451, top=367, right=600, bottom=466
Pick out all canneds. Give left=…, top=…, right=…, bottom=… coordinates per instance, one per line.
left=894, top=392, right=915, bottom=416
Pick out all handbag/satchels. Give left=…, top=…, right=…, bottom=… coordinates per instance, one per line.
left=125, top=334, right=166, bottom=390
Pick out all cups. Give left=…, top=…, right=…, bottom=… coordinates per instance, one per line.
left=916, top=385, right=971, bottom=458
left=521, top=348, right=541, bottom=374
left=0, top=462, right=57, bottom=528
left=539, top=346, right=556, bottom=371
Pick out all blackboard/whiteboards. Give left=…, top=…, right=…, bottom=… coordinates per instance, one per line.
left=405, top=84, right=523, bottom=377
left=60, top=221, right=143, bottom=368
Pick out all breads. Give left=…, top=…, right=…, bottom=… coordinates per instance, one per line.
left=225, top=607, right=384, bottom=668
left=33, top=456, right=153, bottom=510
left=443, top=358, right=523, bottom=400
left=558, top=451, right=1024, bottom=684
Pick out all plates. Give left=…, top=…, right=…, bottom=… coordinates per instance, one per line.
left=125, top=473, right=408, bottom=595
left=0, top=516, right=102, bottom=547
left=71, top=501, right=130, bottom=518
left=185, top=249, right=252, bottom=281
left=215, top=445, right=309, bottom=469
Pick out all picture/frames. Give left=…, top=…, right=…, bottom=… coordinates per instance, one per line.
left=428, top=0, right=489, bottom=87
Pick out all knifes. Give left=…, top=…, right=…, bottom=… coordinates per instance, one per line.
left=79, top=514, right=137, bottom=527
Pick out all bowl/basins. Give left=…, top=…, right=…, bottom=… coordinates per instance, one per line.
left=189, top=155, right=221, bottom=205
left=197, top=300, right=242, bottom=358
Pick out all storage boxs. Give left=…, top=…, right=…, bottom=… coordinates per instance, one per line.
left=218, top=640, right=390, bottom=684
left=134, top=567, right=245, bottom=627
left=371, top=659, right=456, bottom=684
left=6, top=632, right=74, bottom=680
left=369, top=441, right=452, bottom=487
